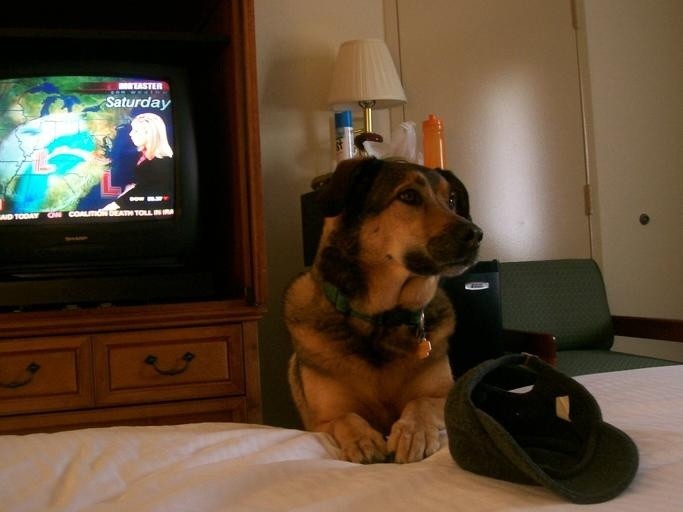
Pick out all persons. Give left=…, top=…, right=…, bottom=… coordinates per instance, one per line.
left=89, top=112, right=173, bottom=208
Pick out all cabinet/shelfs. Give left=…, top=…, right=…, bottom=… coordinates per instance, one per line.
left=0, top=0, right=267, bottom=436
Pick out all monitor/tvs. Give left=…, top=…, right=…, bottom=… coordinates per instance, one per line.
left=0, top=55, right=232, bottom=306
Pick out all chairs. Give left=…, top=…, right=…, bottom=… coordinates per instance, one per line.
left=444, top=258, right=683, bottom=379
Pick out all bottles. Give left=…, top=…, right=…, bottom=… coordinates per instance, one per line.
left=333, top=109, right=356, bottom=162
left=421, top=114, right=446, bottom=172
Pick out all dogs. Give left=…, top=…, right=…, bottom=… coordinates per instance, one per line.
left=282, top=153, right=485, bottom=466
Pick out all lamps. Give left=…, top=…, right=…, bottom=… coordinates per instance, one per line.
left=328, top=37, right=407, bottom=159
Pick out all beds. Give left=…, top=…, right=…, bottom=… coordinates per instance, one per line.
left=0, top=362, right=683, bottom=512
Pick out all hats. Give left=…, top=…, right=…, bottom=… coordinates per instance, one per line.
left=444, top=352, right=638, bottom=504
